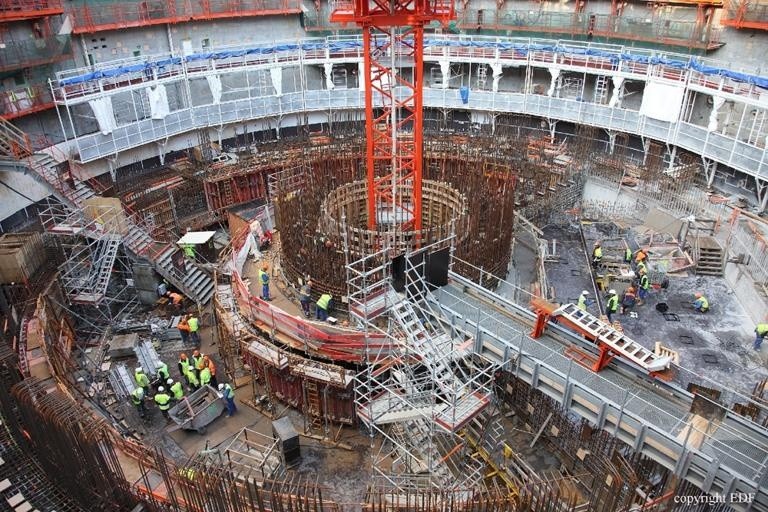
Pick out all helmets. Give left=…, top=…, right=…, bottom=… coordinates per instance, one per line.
left=263, top=265, right=267, bottom=269
left=627, top=287, right=633, bottom=293
left=217, top=383, right=224, bottom=391
left=167, top=292, right=170, bottom=295
left=694, top=292, right=700, bottom=297
left=135, top=362, right=173, bottom=395
left=306, top=280, right=312, bottom=286
left=189, top=314, right=192, bottom=317
left=608, top=289, right=615, bottom=295
left=182, top=319, right=186, bottom=323
left=180, top=350, right=208, bottom=371
left=582, top=290, right=588, bottom=295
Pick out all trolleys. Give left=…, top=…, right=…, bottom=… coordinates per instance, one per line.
left=166, top=384, right=225, bottom=435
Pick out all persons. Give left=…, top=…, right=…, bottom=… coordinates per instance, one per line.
left=300, top=280, right=313, bottom=318
left=315, top=291, right=335, bottom=320
left=754, top=312, right=768, bottom=350
left=578, top=290, right=595, bottom=312
left=259, top=265, right=272, bottom=302
left=131, top=312, right=238, bottom=424
left=166, top=290, right=184, bottom=309
left=689, top=292, right=708, bottom=313
left=592, top=241, right=650, bottom=323
